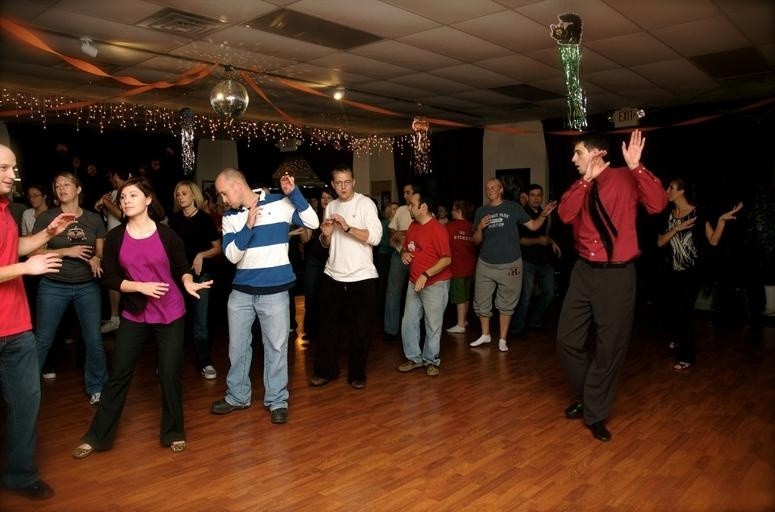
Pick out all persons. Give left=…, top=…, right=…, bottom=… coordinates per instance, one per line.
left=1, top=144, right=78, bottom=501
left=68, top=179, right=214, bottom=459
left=650, top=174, right=744, bottom=371
left=7, top=164, right=562, bottom=425
left=553, top=127, right=669, bottom=441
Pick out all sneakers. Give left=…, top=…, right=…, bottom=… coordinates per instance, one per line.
left=201, top=365, right=217, bottom=379
left=446, top=326, right=465, bottom=333
left=427, top=365, right=439, bottom=375
left=399, top=361, right=423, bottom=371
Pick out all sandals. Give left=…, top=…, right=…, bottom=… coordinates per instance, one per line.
left=673, top=362, right=691, bottom=370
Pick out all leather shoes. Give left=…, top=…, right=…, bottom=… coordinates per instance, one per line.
left=72, top=446, right=93, bottom=458
left=271, top=408, right=288, bottom=423
left=351, top=381, right=364, bottom=389
left=566, top=402, right=584, bottom=418
left=590, top=422, right=611, bottom=440
left=211, top=400, right=245, bottom=413
left=310, top=376, right=329, bottom=387
left=170, top=441, right=186, bottom=452
left=14, top=479, right=53, bottom=499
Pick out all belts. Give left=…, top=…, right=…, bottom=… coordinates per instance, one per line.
left=580, top=256, right=635, bottom=268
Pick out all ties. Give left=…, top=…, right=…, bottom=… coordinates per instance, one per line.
left=588, top=181, right=617, bottom=263
